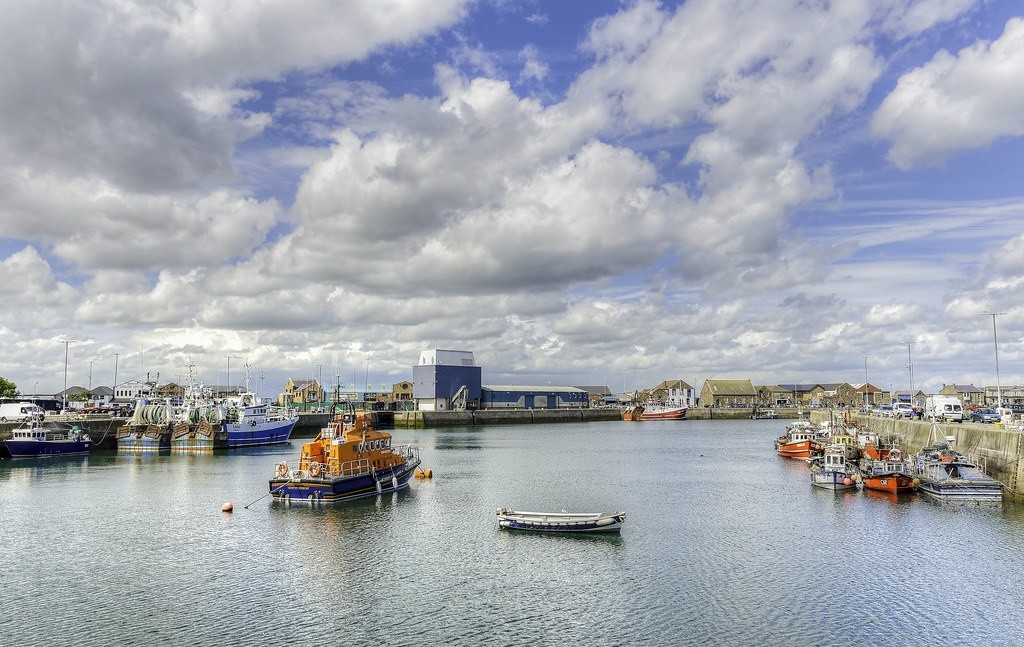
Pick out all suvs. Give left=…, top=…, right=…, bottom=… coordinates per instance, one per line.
left=892, top=400, right=915, bottom=419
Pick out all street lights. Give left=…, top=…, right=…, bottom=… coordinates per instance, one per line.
left=335, top=373, right=342, bottom=403
left=858, top=352, right=874, bottom=410
left=221, top=354, right=236, bottom=397
left=980, top=308, right=1009, bottom=424
left=901, top=340, right=918, bottom=415
left=791, top=375, right=801, bottom=412
left=58, top=339, right=76, bottom=412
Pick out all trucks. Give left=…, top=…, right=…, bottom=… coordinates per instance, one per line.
left=925, top=395, right=963, bottom=421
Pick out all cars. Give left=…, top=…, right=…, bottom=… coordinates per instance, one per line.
left=973, top=406, right=1001, bottom=423
left=878, top=404, right=894, bottom=413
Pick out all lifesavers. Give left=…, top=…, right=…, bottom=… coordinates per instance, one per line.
left=251, top=420, right=256, bottom=426
left=308, top=460, right=321, bottom=478
left=887, top=448, right=902, bottom=462
left=277, top=462, right=288, bottom=476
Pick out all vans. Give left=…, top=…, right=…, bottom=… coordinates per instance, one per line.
left=0, top=401, right=44, bottom=421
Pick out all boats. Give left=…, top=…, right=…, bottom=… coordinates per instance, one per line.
left=3, top=403, right=94, bottom=459
left=907, top=424, right=1003, bottom=503
left=265, top=400, right=422, bottom=504
left=809, top=453, right=861, bottom=493
left=859, top=452, right=917, bottom=496
left=638, top=405, right=688, bottom=421
left=494, top=505, right=627, bottom=538
left=112, top=358, right=301, bottom=450
left=775, top=409, right=906, bottom=461
left=858, top=427, right=897, bottom=459
